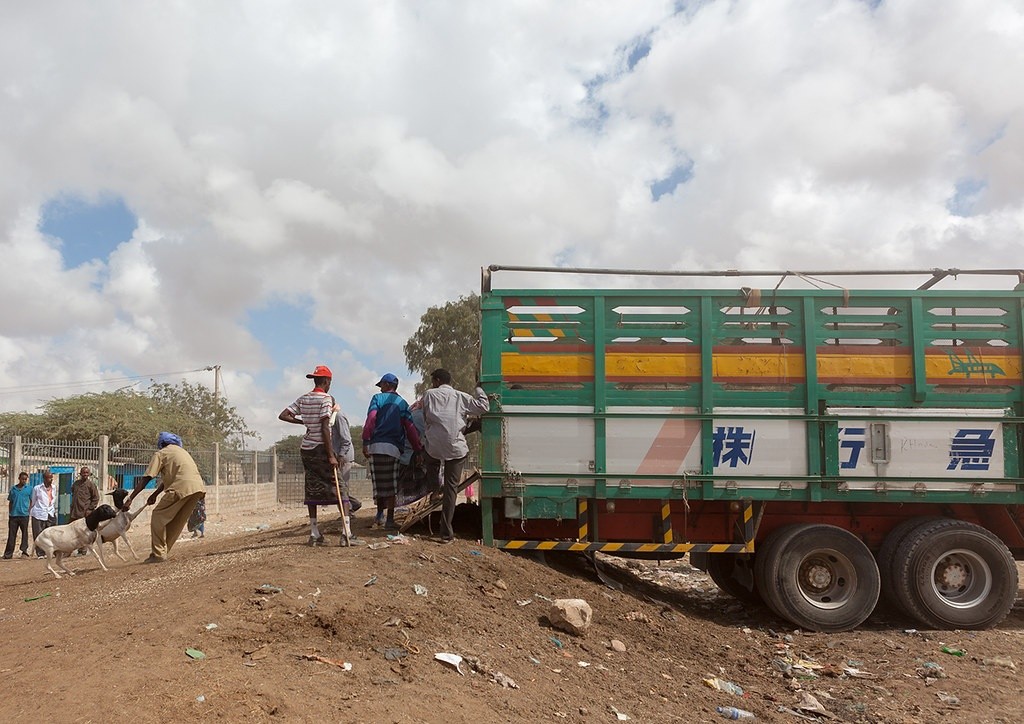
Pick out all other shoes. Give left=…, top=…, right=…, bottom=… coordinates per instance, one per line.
left=1, top=555, right=12, bottom=560
left=442, top=536, right=458, bottom=543
left=375, top=515, right=386, bottom=525
left=192, top=533, right=198, bottom=538
left=430, top=492, right=443, bottom=502
left=200, top=534, right=204, bottom=538
left=22, top=552, right=29, bottom=557
left=38, top=554, right=48, bottom=559
left=309, top=533, right=331, bottom=546
left=144, top=555, right=164, bottom=564
left=340, top=534, right=366, bottom=547
left=77, top=551, right=86, bottom=555
left=384, top=521, right=402, bottom=530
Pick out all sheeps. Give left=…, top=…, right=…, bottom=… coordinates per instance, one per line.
left=96, top=488, right=148, bottom=566
left=31, top=504, right=117, bottom=579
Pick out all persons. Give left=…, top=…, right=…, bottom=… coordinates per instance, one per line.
left=191, top=497, right=206, bottom=538
left=279, top=366, right=366, bottom=546
left=330, top=396, right=357, bottom=520
left=122, top=432, right=207, bottom=564
left=63, top=467, right=100, bottom=558
left=362, top=368, right=490, bottom=544
left=31, top=472, right=57, bottom=559
left=0, top=472, right=32, bottom=559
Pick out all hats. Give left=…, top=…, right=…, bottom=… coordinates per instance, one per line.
left=375, top=373, right=398, bottom=388
left=306, top=365, right=332, bottom=379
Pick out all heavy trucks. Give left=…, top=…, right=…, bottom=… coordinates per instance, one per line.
left=464, top=264, right=1022, bottom=633
